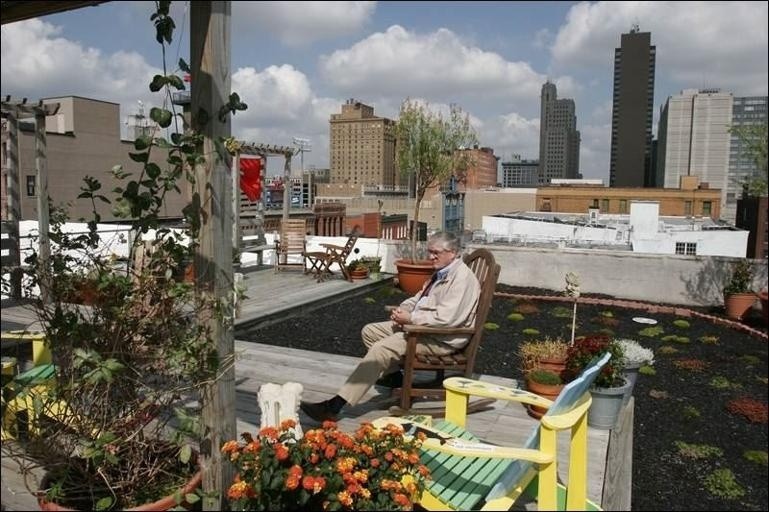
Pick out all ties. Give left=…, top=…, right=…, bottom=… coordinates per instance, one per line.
left=418, top=273, right=437, bottom=301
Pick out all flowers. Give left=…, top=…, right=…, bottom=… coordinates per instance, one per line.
left=221, top=417, right=431, bottom=512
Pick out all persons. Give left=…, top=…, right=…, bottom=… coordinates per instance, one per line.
left=300, top=231, right=482, bottom=423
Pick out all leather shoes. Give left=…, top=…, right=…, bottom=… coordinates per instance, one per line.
left=375, top=373, right=404, bottom=388
left=300, top=400, right=340, bottom=422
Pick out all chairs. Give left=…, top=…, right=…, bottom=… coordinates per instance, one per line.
left=384, top=247, right=502, bottom=412
left=273, top=219, right=308, bottom=274
left=367, top=350, right=613, bottom=512
left=314, top=224, right=362, bottom=283
left=1, top=328, right=61, bottom=442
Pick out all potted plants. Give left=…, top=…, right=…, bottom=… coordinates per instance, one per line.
left=383, top=94, right=479, bottom=294
left=525, top=369, right=563, bottom=419
left=723, top=258, right=758, bottom=323
left=363, top=256, right=381, bottom=273
left=518, top=338, right=569, bottom=373
left=617, top=338, right=654, bottom=395
left=724, top=122, right=768, bottom=325
left=345, top=262, right=369, bottom=279
left=0, top=0, right=247, bottom=512
left=567, top=334, right=631, bottom=430
